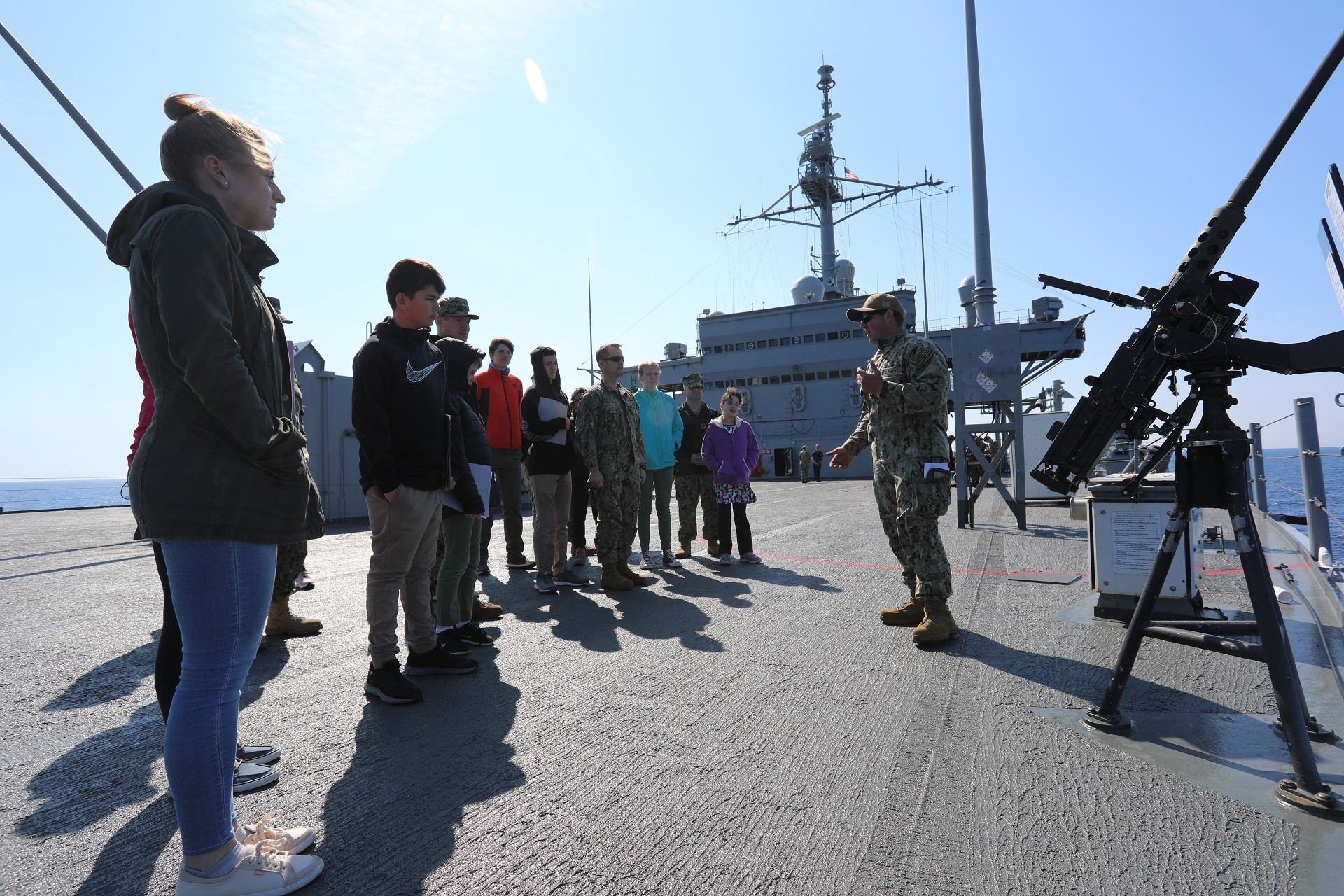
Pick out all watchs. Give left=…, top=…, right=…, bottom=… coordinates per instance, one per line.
left=640, top=465, right=646, bottom=471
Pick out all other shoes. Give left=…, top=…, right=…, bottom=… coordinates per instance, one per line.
left=880, top=596, right=955, bottom=643
left=477, top=561, right=491, bottom=576
left=505, top=553, right=537, bottom=568
left=472, top=597, right=503, bottom=618
left=571, top=545, right=596, bottom=565
left=719, top=552, right=762, bottom=565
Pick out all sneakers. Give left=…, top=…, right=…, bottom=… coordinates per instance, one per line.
left=534, top=574, right=558, bottom=594
left=552, top=567, right=589, bottom=586
left=640, top=548, right=682, bottom=570
left=364, top=659, right=423, bottom=705
left=404, top=643, right=479, bottom=676
left=176, top=837, right=324, bottom=896
left=167, top=741, right=280, bottom=798
left=439, top=623, right=494, bottom=655
left=241, top=809, right=317, bottom=854
left=294, top=571, right=315, bottom=589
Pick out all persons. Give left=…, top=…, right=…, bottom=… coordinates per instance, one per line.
left=799, top=446, right=811, bottom=483
left=428, top=297, right=502, bottom=655
left=107, top=93, right=324, bottom=896
left=826, top=293, right=958, bottom=643
left=128, top=296, right=281, bottom=800
left=568, top=387, right=597, bottom=567
left=811, top=444, right=823, bottom=483
left=574, top=344, right=647, bottom=590
left=257, top=296, right=324, bottom=650
left=786, top=451, right=792, bottom=474
left=633, top=359, right=684, bottom=569
left=474, top=337, right=536, bottom=574
left=702, top=386, right=762, bottom=565
left=673, top=374, right=720, bottom=559
left=352, top=259, right=479, bottom=703
left=948, top=435, right=1001, bottom=487
left=520, top=347, right=589, bottom=591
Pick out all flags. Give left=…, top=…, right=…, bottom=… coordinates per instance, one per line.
left=844, top=167, right=858, bottom=181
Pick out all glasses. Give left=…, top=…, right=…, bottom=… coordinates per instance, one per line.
left=861, top=310, right=886, bottom=323
left=602, top=356, right=624, bottom=362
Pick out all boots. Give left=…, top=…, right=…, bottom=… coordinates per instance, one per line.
left=600, top=560, right=648, bottom=591
left=259, top=594, right=323, bottom=650
left=675, top=538, right=720, bottom=560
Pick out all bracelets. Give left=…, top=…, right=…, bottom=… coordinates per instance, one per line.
left=879, top=380, right=888, bottom=398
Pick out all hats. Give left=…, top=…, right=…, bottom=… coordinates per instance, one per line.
left=846, top=293, right=905, bottom=322
left=437, top=297, right=480, bottom=320
left=681, top=374, right=704, bottom=389
left=267, top=296, right=293, bottom=324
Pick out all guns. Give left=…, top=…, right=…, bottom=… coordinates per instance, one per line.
left=1028, top=29, right=1344, bottom=499
